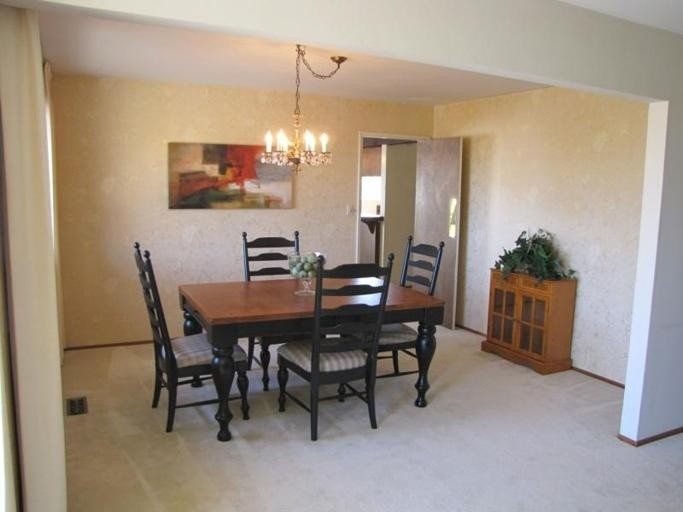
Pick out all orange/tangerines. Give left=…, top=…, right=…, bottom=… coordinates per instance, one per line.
left=291, top=252, right=318, bottom=278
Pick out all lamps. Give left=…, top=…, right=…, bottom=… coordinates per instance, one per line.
left=360, top=172, right=386, bottom=267
left=259, top=43, right=348, bottom=176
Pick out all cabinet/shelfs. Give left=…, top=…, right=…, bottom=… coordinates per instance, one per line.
left=480, top=267, right=578, bottom=375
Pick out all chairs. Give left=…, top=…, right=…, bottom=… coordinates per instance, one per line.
left=130, top=240, right=249, bottom=434
left=275, top=252, right=394, bottom=442
left=338, top=234, right=445, bottom=378
left=240, top=227, right=326, bottom=391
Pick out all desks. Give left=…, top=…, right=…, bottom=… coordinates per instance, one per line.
left=177, top=276, right=446, bottom=442
